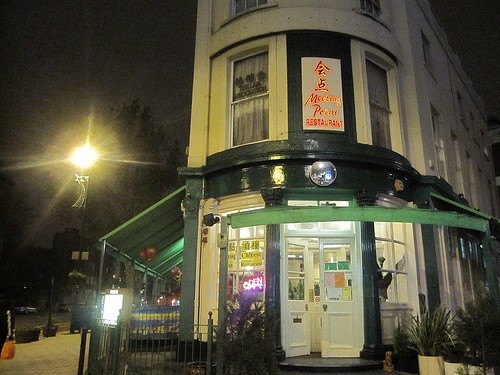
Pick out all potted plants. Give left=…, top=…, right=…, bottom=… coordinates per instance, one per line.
left=398, top=293, right=500, bottom=374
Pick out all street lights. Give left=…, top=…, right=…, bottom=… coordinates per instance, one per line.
left=66, top=135, right=97, bottom=335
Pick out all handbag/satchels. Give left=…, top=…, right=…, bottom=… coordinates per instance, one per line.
left=0, top=335, right=15, bottom=361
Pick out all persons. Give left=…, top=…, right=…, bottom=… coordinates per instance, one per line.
left=0, top=294, right=17, bottom=355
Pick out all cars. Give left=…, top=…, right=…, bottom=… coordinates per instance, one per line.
left=14, top=303, right=38, bottom=315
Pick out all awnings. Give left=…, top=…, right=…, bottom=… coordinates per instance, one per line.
left=224, top=208, right=489, bottom=241
left=98, top=185, right=185, bottom=281
left=433, top=193, right=500, bottom=242
